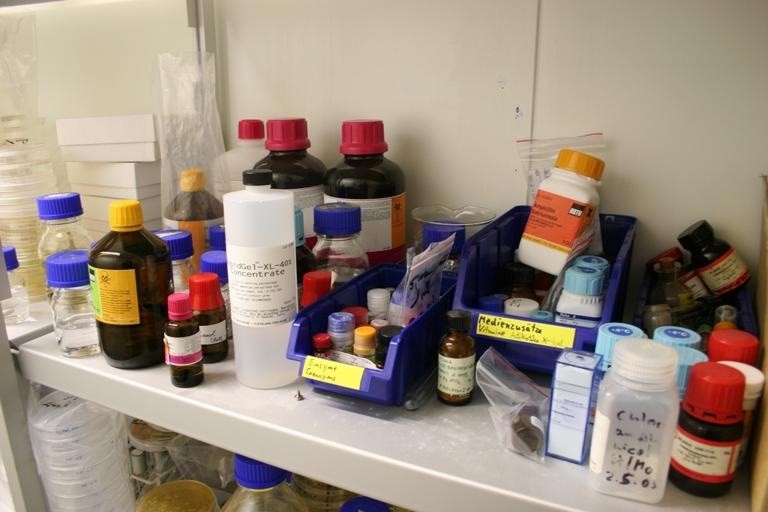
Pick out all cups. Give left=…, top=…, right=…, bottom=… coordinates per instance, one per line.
left=412, top=203, right=495, bottom=250
left=135, top=479, right=217, bottom=511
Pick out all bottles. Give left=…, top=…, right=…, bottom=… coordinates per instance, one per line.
left=421, top=218, right=466, bottom=294
left=38, top=114, right=409, bottom=390
left=432, top=308, right=479, bottom=406
left=220, top=452, right=388, bottom=512
left=312, top=283, right=414, bottom=368
left=588, top=219, right=765, bottom=504
left=0, top=246, right=29, bottom=326
left=480, top=148, right=610, bottom=329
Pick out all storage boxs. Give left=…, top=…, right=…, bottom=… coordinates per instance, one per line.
left=452, top=199, right=639, bottom=378
left=280, top=263, right=459, bottom=408
